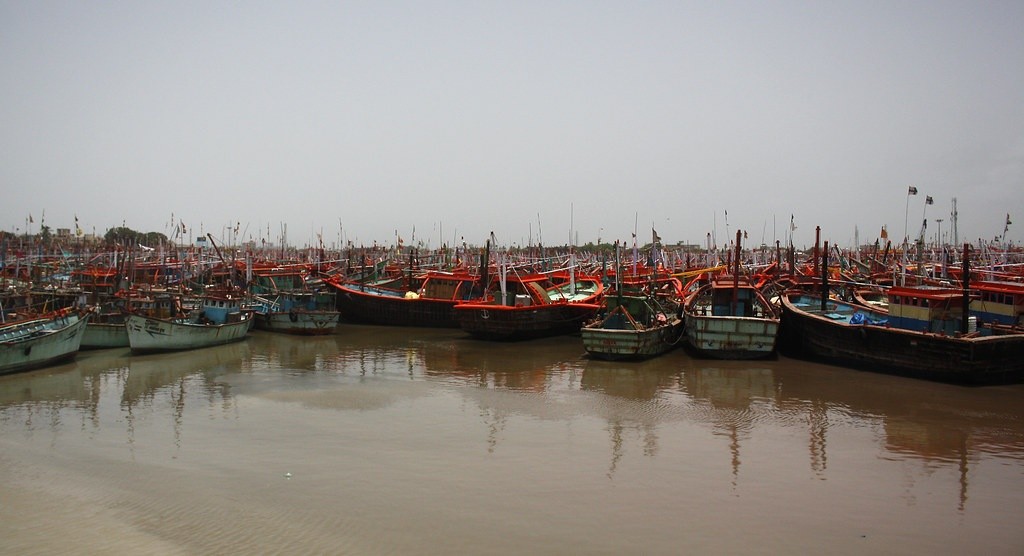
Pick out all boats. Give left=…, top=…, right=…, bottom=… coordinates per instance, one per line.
left=0, top=185, right=1024, bottom=384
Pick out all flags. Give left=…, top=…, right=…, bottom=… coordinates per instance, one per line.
left=926, top=196, right=934, bottom=205
left=182, top=223, right=185, bottom=228
left=653, top=229, right=661, bottom=243
left=908, top=186, right=917, bottom=195
left=30, top=216, right=33, bottom=223
left=183, top=229, right=186, bottom=233
left=881, top=229, right=888, bottom=238
left=923, top=219, right=927, bottom=229
left=176, top=226, right=180, bottom=238
